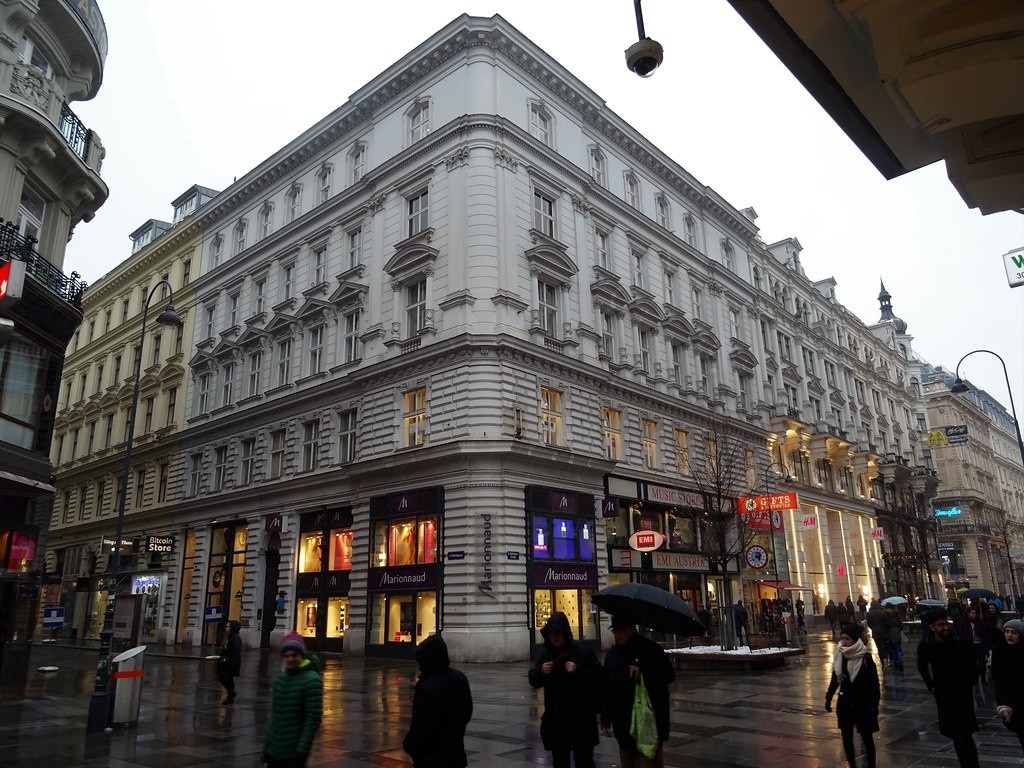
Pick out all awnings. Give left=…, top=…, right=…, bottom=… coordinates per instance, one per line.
left=756, top=581, right=814, bottom=591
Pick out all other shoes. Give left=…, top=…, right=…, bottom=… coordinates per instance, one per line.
left=222, top=692, right=237, bottom=705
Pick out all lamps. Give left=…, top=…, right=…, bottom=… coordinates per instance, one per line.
left=21, top=234, right=34, bottom=261
left=26, top=237, right=39, bottom=267
left=71, top=274, right=81, bottom=301
left=67, top=270, right=77, bottom=300
left=1, top=220, right=12, bottom=253
left=6, top=224, right=20, bottom=261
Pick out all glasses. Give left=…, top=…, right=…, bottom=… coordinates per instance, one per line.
left=935, top=622, right=949, bottom=627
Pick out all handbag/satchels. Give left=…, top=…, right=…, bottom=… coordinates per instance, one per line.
left=629, top=672, right=658, bottom=760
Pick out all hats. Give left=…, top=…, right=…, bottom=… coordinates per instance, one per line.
left=840, top=624, right=864, bottom=642
left=608, top=613, right=635, bottom=629
left=1003, top=618, right=1024, bottom=635
left=279, top=631, right=305, bottom=656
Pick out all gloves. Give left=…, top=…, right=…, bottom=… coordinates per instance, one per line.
left=825, top=700, right=832, bottom=712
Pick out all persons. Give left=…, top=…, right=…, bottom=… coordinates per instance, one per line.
left=824, top=595, right=866, bottom=632
left=731, top=600, right=749, bottom=645
left=825, top=624, right=880, bottom=768
left=600, top=612, right=675, bottom=768
left=697, top=604, right=711, bottom=637
left=867, top=597, right=905, bottom=674
left=260, top=631, right=323, bottom=768
left=990, top=618, right=1024, bottom=753
left=916, top=608, right=981, bottom=768
left=217, top=620, right=242, bottom=705
left=402, top=634, right=474, bottom=768
left=947, top=594, right=1024, bottom=694
left=758, top=600, right=808, bottom=636
left=528, top=612, right=603, bottom=768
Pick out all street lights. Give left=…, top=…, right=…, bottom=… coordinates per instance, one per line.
left=950, top=349, right=1024, bottom=467
left=764, top=461, right=796, bottom=603
left=82, top=278, right=182, bottom=733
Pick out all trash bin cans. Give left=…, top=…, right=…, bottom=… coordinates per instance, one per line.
left=106, top=646, right=147, bottom=728
left=72, top=628, right=78, bottom=642
left=782, top=612, right=793, bottom=645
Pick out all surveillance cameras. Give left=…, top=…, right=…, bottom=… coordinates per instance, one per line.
left=625, top=40, right=664, bottom=78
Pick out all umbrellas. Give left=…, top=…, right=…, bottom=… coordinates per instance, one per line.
left=881, top=596, right=908, bottom=606
left=917, top=599, right=945, bottom=608
left=957, top=587, right=969, bottom=593
left=591, top=582, right=706, bottom=680
left=960, top=588, right=997, bottom=598
